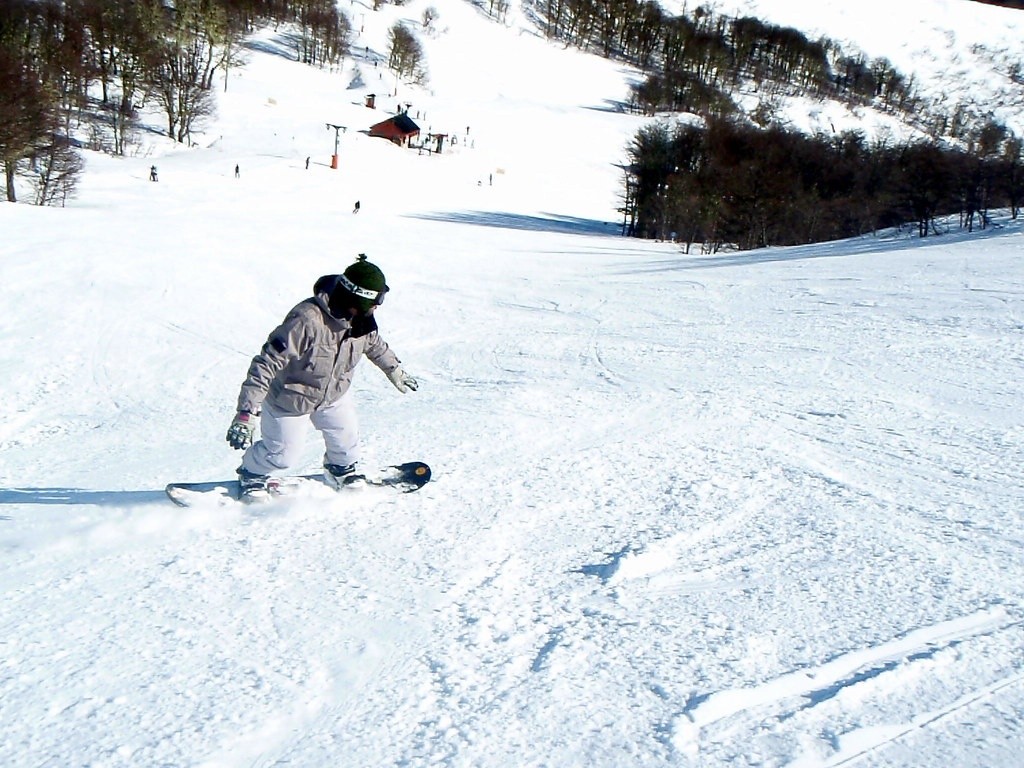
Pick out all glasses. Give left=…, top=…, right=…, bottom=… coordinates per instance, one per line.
left=374, top=286, right=387, bottom=306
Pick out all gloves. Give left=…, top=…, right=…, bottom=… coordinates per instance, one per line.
left=386, top=365, right=419, bottom=393
left=226, top=410, right=253, bottom=450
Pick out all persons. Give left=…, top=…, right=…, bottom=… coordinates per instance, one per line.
left=225, top=262, right=419, bottom=503
left=150, top=165, right=157, bottom=181
left=305, top=156, right=310, bottom=167
left=235, top=164, right=239, bottom=178
left=353, top=201, right=361, bottom=214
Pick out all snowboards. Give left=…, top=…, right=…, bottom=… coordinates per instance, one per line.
left=165, top=461, right=432, bottom=508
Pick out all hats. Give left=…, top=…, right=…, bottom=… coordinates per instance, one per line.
left=331, top=254, right=390, bottom=315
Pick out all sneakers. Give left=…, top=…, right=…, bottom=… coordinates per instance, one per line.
left=323, top=461, right=370, bottom=493
left=236, top=466, right=271, bottom=506
